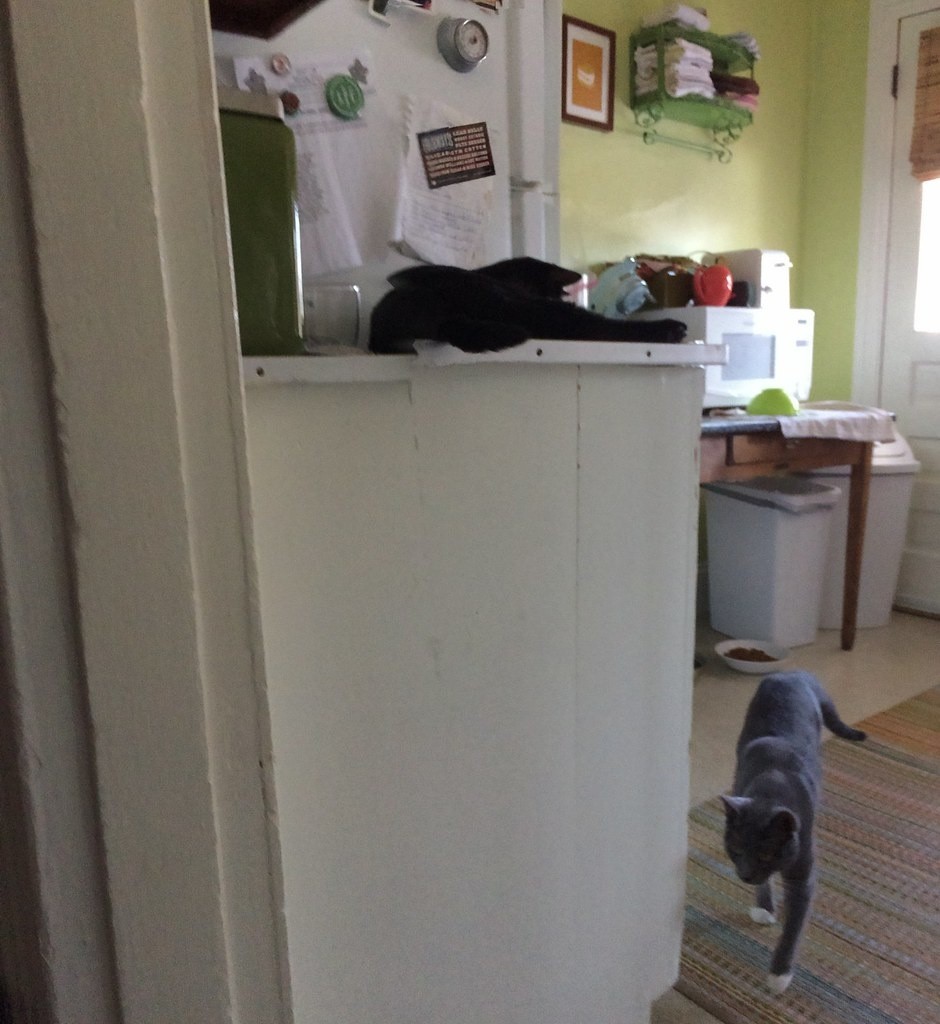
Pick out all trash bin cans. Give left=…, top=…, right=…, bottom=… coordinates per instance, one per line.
left=809, top=430, right=922, bottom=630
left=701, top=473, right=845, bottom=651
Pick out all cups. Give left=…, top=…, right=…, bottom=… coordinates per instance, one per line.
left=302, top=281, right=362, bottom=357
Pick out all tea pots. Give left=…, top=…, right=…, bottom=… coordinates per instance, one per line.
left=691, top=260, right=733, bottom=307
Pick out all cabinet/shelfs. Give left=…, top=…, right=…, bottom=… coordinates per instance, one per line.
left=629, top=17, right=759, bottom=163
left=242, top=343, right=732, bottom=1024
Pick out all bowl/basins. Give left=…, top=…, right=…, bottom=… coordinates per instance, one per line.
left=714, top=640, right=793, bottom=674
left=748, top=387, right=798, bottom=417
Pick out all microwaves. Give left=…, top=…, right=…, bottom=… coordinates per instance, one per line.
left=650, top=303, right=817, bottom=408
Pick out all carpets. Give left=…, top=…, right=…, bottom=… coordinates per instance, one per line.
left=671, top=684, right=940, bottom=1024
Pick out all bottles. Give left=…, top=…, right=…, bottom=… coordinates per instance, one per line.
left=216, top=84, right=303, bottom=354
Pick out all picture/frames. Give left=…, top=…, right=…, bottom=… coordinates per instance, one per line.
left=559, top=13, right=617, bottom=133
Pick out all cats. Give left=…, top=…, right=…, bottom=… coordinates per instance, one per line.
left=718, top=672, right=867, bottom=997
left=367, top=254, right=689, bottom=358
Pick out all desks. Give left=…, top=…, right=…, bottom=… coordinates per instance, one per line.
left=697, top=400, right=898, bottom=652
